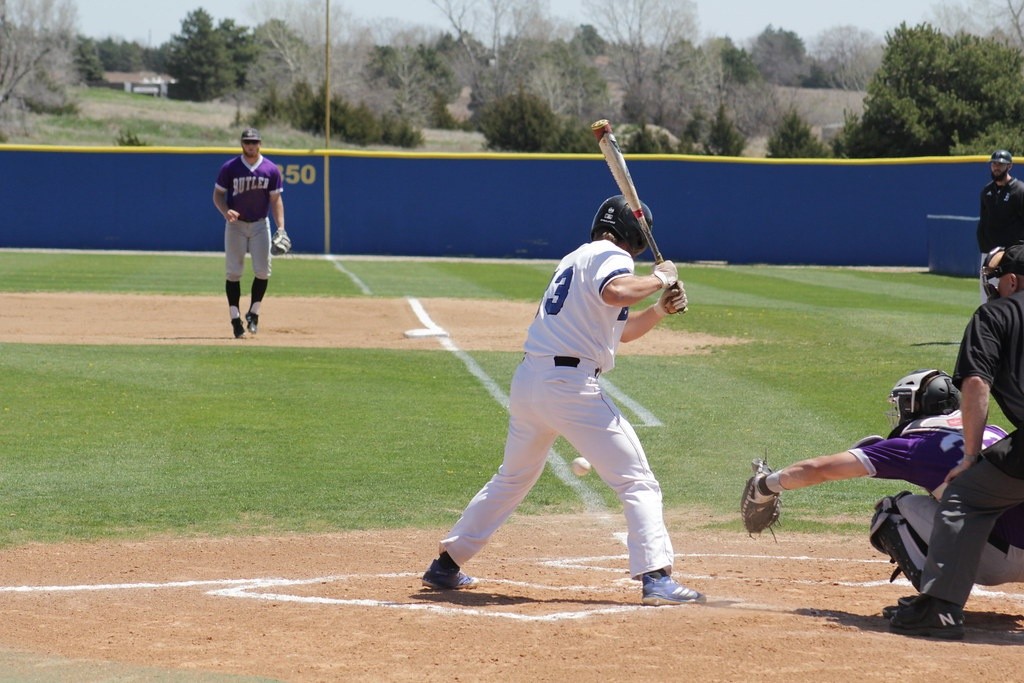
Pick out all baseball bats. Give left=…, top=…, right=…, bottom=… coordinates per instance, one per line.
left=591, top=118, right=689, bottom=315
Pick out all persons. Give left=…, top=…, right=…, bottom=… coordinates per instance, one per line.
left=882, top=244, right=1024, bottom=639
left=976, top=152, right=1024, bottom=304
left=422, top=194, right=707, bottom=606
left=741, top=369, right=1024, bottom=620
left=213, top=129, right=291, bottom=338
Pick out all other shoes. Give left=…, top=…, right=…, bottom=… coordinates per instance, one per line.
left=232, top=318, right=245, bottom=337
left=246, top=312, right=259, bottom=333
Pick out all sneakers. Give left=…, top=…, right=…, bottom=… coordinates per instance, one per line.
left=889, top=598, right=964, bottom=638
left=642, top=574, right=707, bottom=605
left=423, top=559, right=479, bottom=589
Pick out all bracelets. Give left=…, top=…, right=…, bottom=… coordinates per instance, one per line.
left=964, top=453, right=975, bottom=463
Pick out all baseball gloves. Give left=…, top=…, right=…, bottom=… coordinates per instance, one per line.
left=739, top=458, right=782, bottom=533
left=270, top=228, right=291, bottom=256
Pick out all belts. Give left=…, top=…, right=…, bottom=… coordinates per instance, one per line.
left=555, top=356, right=580, bottom=367
left=988, top=534, right=1009, bottom=553
left=238, top=216, right=258, bottom=222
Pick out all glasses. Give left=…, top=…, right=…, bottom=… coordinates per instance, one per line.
left=244, top=140, right=259, bottom=144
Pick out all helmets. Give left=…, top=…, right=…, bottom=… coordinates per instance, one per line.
left=990, top=150, right=1011, bottom=164
left=591, top=195, right=653, bottom=253
left=885, top=369, right=960, bottom=429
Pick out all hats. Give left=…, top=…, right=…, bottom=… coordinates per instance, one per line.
left=241, top=129, right=261, bottom=140
left=997, top=245, right=1024, bottom=276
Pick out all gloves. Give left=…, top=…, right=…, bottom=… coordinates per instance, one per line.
left=655, top=281, right=688, bottom=317
left=654, top=260, right=678, bottom=288
